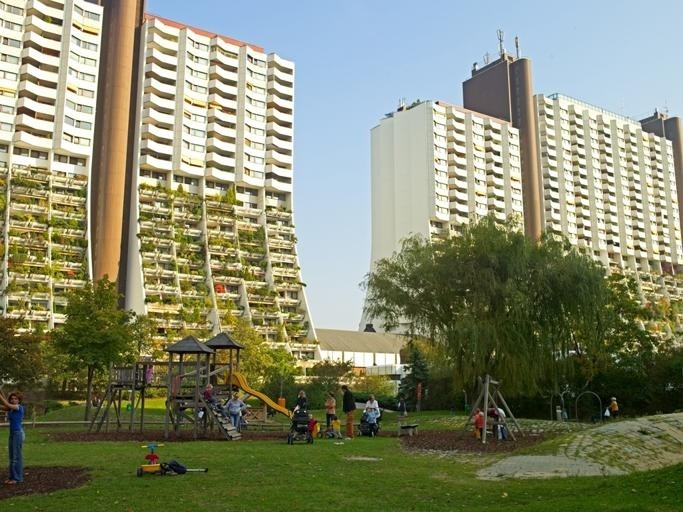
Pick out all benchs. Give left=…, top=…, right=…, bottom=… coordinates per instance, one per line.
left=397, top=416, right=419, bottom=437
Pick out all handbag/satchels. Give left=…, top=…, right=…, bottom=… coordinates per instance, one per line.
left=604, top=408, right=610, bottom=416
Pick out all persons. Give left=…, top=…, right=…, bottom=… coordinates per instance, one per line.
left=0, top=392, right=24, bottom=484
left=487, top=408, right=508, bottom=441
left=341, top=386, right=355, bottom=440
left=297, top=390, right=307, bottom=414
left=606, top=397, right=619, bottom=419
left=472, top=407, right=484, bottom=440
left=201, top=384, right=245, bottom=431
left=324, top=393, right=343, bottom=438
left=146, top=365, right=154, bottom=386
left=364, top=394, right=381, bottom=437
left=398, top=398, right=408, bottom=418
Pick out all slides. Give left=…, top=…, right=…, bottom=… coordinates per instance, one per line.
left=231, top=371, right=292, bottom=417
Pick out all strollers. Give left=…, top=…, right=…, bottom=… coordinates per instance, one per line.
left=288, top=410, right=313, bottom=445
left=356, top=407, right=383, bottom=438
